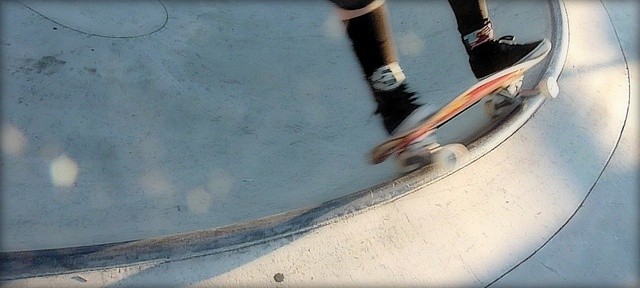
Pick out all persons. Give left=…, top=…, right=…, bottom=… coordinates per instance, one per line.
left=330, top=0, right=552, bottom=136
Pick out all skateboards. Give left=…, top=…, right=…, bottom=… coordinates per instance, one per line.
left=366, top=39, right=559, bottom=173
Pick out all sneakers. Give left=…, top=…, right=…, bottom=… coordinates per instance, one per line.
left=469, top=36, right=551, bottom=82
left=375, top=91, right=441, bottom=136
